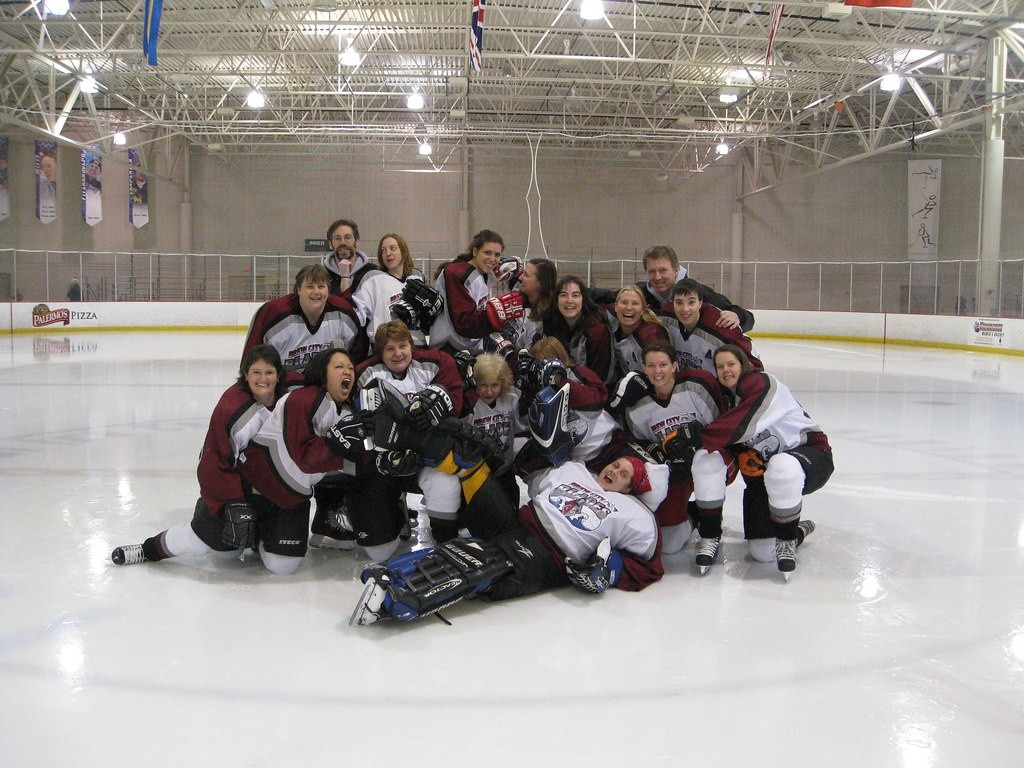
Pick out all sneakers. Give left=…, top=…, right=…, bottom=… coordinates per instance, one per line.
left=348, top=574, right=386, bottom=629
left=104, top=536, right=149, bottom=566
left=772, top=536, right=799, bottom=581
left=793, top=520, right=816, bottom=549
left=360, top=387, right=384, bottom=452
left=399, top=490, right=419, bottom=540
left=695, top=527, right=723, bottom=575
left=309, top=503, right=357, bottom=549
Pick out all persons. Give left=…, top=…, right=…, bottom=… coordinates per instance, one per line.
left=112, top=218, right=444, bottom=575
left=132, top=163, right=148, bottom=204
left=429, top=229, right=617, bottom=389
left=85, top=157, right=102, bottom=194
left=971, top=298, right=975, bottom=313
left=518, top=336, right=665, bottom=592
left=41, top=149, right=56, bottom=195
left=13, top=287, right=24, bottom=302
left=418, top=435, right=665, bottom=603
left=67, top=278, right=84, bottom=302
left=352, top=318, right=531, bottom=564
left=955, top=296, right=967, bottom=314
left=0, top=156, right=8, bottom=185
left=591, top=247, right=835, bottom=572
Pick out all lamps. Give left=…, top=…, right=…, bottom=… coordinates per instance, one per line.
left=419, top=137, right=431, bottom=154
left=121, top=34, right=141, bottom=64
left=769, top=60, right=787, bottom=80
left=406, top=85, right=423, bottom=111
left=113, top=130, right=126, bottom=146
left=314, top=0, right=337, bottom=12
left=556, top=39, right=578, bottom=69
left=80, top=63, right=99, bottom=93
left=656, top=163, right=667, bottom=181
left=338, top=33, right=361, bottom=66
left=719, top=78, right=738, bottom=102
left=715, top=136, right=729, bottom=155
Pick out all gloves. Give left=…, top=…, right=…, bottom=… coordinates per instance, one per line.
left=492, top=253, right=524, bottom=282
left=645, top=442, right=685, bottom=482
left=393, top=276, right=444, bottom=329
left=733, top=444, right=767, bottom=476
left=606, top=371, right=652, bottom=408
left=486, top=291, right=523, bottom=330
left=405, top=383, right=454, bottom=433
left=533, top=356, right=565, bottom=389
left=220, top=501, right=259, bottom=557
left=663, top=419, right=703, bottom=458
left=453, top=349, right=476, bottom=389
left=369, top=446, right=423, bottom=478
left=518, top=347, right=534, bottom=390
left=325, top=408, right=375, bottom=453
